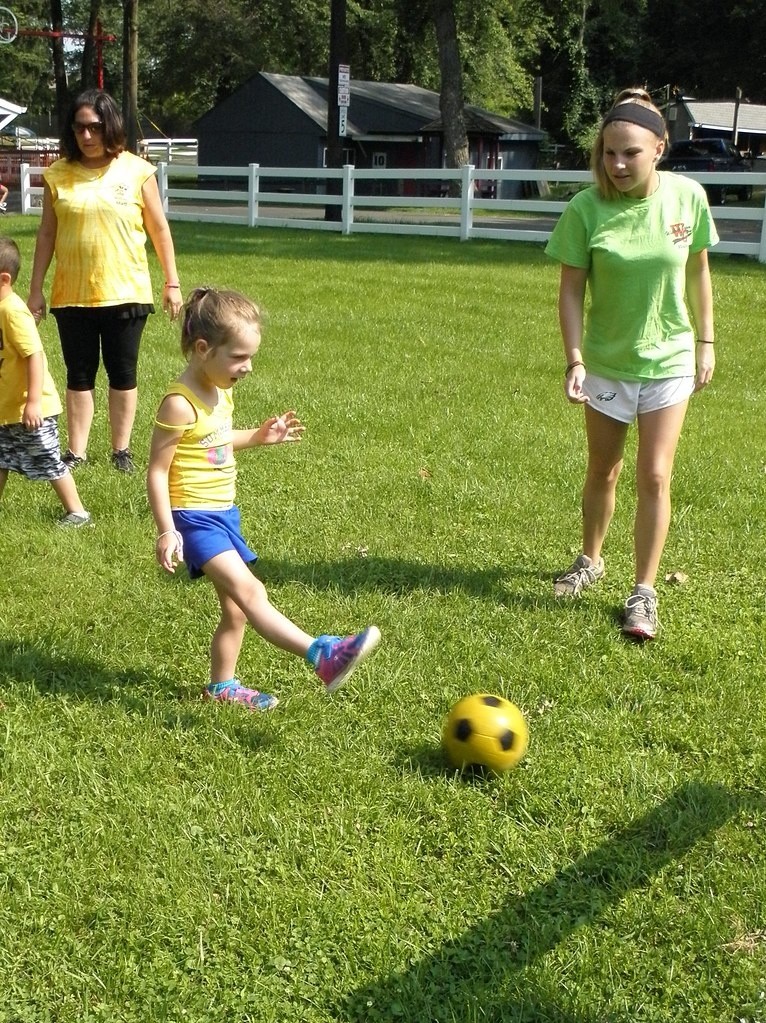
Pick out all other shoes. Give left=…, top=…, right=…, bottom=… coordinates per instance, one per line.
left=0, top=202, right=7, bottom=210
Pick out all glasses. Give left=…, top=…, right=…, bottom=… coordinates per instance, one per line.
left=70, top=121, right=104, bottom=134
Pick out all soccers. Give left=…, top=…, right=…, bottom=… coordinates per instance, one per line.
left=440, top=691, right=529, bottom=777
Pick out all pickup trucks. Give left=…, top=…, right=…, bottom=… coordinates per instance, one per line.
left=657, top=139, right=754, bottom=204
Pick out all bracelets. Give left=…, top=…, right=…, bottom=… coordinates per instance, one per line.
left=165, top=281, right=180, bottom=288
left=697, top=340, right=714, bottom=344
left=565, top=361, right=586, bottom=378
left=157, top=529, right=183, bottom=552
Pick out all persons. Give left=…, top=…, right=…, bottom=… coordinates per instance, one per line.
left=0, top=237, right=96, bottom=532
left=147, top=286, right=382, bottom=712
left=544, top=88, right=720, bottom=641
left=27, top=88, right=183, bottom=474
left=0, top=184, right=9, bottom=207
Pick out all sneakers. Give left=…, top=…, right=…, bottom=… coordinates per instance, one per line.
left=555, top=556, right=605, bottom=595
left=56, top=512, right=95, bottom=530
left=59, top=448, right=87, bottom=472
left=110, top=448, right=134, bottom=475
left=202, top=680, right=279, bottom=712
left=623, top=583, right=665, bottom=640
left=315, top=626, right=381, bottom=692
